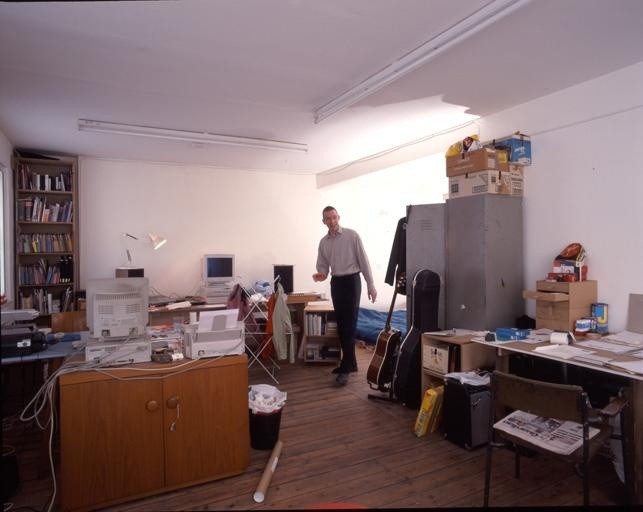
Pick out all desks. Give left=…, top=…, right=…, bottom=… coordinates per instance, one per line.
left=149, top=294, right=321, bottom=354
left=1, top=328, right=85, bottom=364
left=470, top=331, right=643, bottom=506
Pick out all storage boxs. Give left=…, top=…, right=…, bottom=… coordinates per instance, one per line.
left=446, top=138, right=531, bottom=200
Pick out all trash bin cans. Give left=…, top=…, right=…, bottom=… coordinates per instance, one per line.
left=249, top=408, right=283, bottom=450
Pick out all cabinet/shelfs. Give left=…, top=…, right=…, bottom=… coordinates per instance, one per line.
left=303, top=304, right=344, bottom=365
left=421, top=332, right=496, bottom=403
left=406, top=194, right=524, bottom=332
left=13, top=155, right=79, bottom=327
left=521, top=280, right=597, bottom=332
left=59, top=351, right=251, bottom=512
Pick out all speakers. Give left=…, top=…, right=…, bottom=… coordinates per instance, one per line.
left=115, top=268, right=144, bottom=277
left=274, top=265, right=293, bottom=294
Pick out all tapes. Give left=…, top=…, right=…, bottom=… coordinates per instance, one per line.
left=586, top=333, right=601, bottom=339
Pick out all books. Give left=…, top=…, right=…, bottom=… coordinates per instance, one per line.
left=146, top=324, right=185, bottom=343
left=15, top=164, right=76, bottom=314
left=305, top=313, right=341, bottom=361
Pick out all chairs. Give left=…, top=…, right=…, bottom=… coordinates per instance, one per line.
left=52, top=311, right=87, bottom=332
left=483, top=370, right=634, bottom=505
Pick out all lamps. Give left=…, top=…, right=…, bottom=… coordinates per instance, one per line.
left=315, top=0, right=527, bottom=124
left=77, top=119, right=307, bottom=154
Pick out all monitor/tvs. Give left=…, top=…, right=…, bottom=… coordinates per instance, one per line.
left=85, top=277, right=150, bottom=343
left=202, top=254, right=236, bottom=288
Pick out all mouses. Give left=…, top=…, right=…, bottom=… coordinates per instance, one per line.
left=72, top=340, right=82, bottom=351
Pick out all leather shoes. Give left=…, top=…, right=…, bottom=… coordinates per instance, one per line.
left=333, top=360, right=357, bottom=374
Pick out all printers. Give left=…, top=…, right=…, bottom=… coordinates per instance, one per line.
left=181, top=313, right=245, bottom=359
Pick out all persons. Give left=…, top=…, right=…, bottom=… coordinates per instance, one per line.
left=312, top=206, right=378, bottom=385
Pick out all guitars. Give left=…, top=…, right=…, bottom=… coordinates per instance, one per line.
left=367, top=262, right=406, bottom=384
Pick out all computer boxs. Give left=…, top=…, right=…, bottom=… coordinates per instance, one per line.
left=200, top=286, right=236, bottom=303
left=83, top=337, right=152, bottom=367
